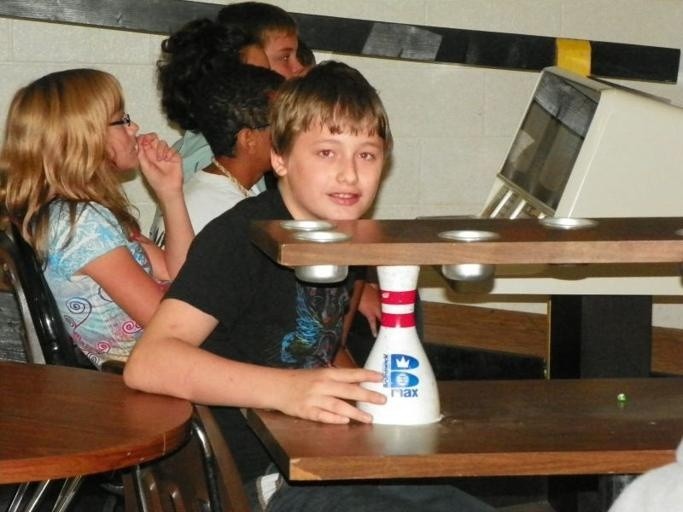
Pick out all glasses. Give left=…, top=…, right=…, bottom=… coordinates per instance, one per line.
left=103, top=112, right=129, bottom=129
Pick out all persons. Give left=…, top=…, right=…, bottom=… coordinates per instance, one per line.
left=150, top=1, right=317, bottom=252
left=123, top=59, right=494, bottom=511
left=1, top=68, right=195, bottom=368
